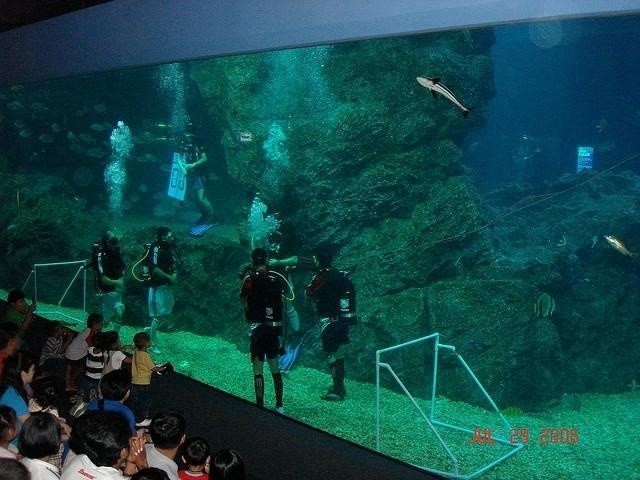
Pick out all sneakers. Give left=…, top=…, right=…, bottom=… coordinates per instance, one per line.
left=274, top=405, right=285, bottom=415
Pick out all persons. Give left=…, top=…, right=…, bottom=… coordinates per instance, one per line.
left=240, top=248, right=289, bottom=412
left=93, top=230, right=125, bottom=334
left=145, top=226, right=178, bottom=355
left=179, top=121, right=214, bottom=236
left=258, top=213, right=302, bottom=372
left=304, top=248, right=350, bottom=402
